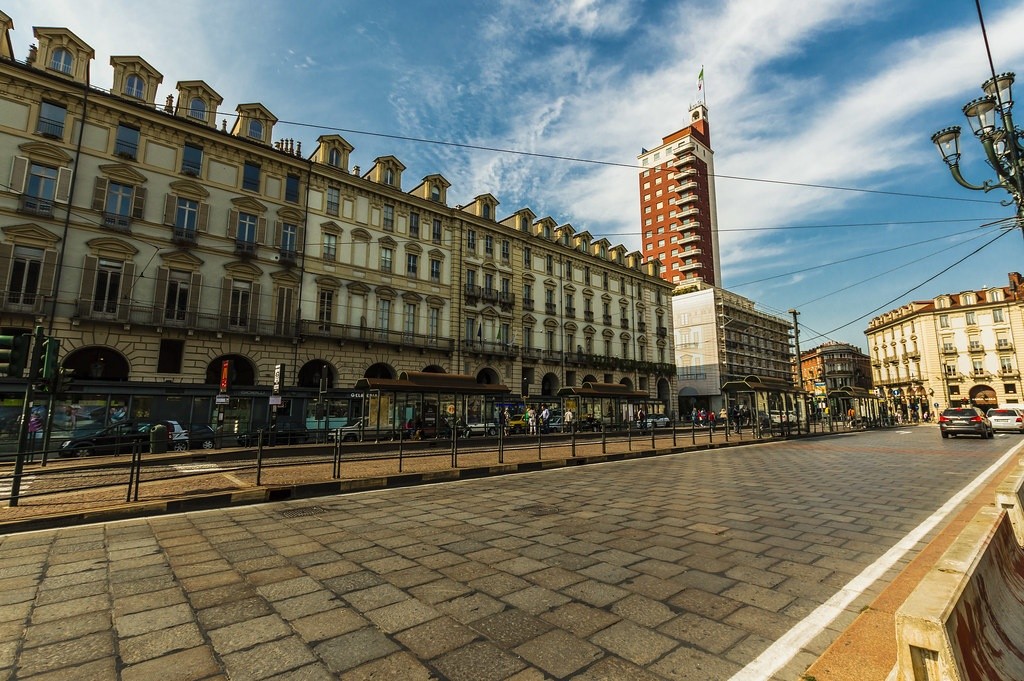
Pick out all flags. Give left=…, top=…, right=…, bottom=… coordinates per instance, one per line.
left=697, top=68, right=704, bottom=90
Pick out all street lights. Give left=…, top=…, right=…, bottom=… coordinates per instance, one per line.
left=788, top=309, right=810, bottom=434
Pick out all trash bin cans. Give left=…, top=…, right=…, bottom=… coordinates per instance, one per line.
left=149, top=424, right=167, bottom=454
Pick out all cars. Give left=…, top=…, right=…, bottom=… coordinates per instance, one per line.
left=137, top=420, right=189, bottom=451
left=508, top=415, right=543, bottom=434
left=573, top=418, right=598, bottom=433
left=179, top=423, right=215, bottom=449
left=940, top=408, right=994, bottom=439
left=237, top=420, right=309, bottom=446
left=637, top=413, right=671, bottom=429
left=548, top=416, right=571, bottom=433
left=328, top=417, right=394, bottom=442
left=770, top=409, right=798, bottom=427
left=986, top=408, right=1024, bottom=434
left=457, top=422, right=497, bottom=435
left=57, top=417, right=175, bottom=457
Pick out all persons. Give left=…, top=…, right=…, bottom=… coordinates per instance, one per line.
left=637, top=409, right=644, bottom=429
left=825, top=407, right=935, bottom=428
left=586, top=414, right=596, bottom=433
left=624, top=419, right=629, bottom=431
left=28, top=414, right=43, bottom=433
left=405, top=404, right=550, bottom=441
left=15, top=416, right=21, bottom=431
left=590, top=414, right=602, bottom=432
left=691, top=404, right=750, bottom=433
left=565, top=408, right=572, bottom=434
